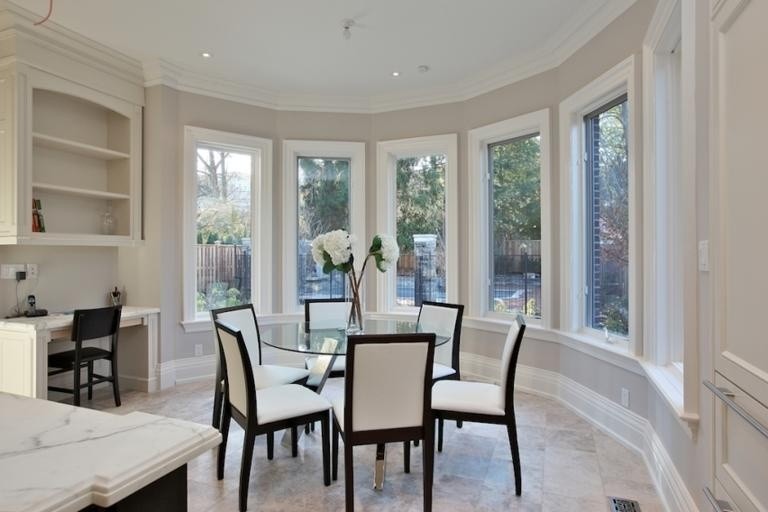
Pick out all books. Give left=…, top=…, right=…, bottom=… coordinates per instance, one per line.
left=31, top=197, right=45, bottom=232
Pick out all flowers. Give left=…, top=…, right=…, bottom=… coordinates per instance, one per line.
left=312, top=229, right=400, bottom=330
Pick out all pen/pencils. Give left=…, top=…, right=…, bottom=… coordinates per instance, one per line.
left=115, top=287, right=117, bottom=295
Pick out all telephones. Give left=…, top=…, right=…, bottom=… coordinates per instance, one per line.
left=24, top=295, right=47, bottom=317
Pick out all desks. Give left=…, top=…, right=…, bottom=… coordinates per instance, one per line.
left=1, top=306, right=160, bottom=400
left=0, top=392, right=223, bottom=512
left=260, top=321, right=452, bottom=450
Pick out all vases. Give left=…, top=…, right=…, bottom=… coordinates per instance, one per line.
left=345, top=271, right=367, bottom=338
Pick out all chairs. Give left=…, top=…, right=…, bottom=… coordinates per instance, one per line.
left=302, top=298, right=359, bottom=432
left=330, top=332, right=437, bottom=512
left=215, top=318, right=333, bottom=512
left=47, top=304, right=122, bottom=407
left=410, top=300, right=465, bottom=445
left=431, top=313, right=526, bottom=496
left=208, top=304, right=310, bottom=459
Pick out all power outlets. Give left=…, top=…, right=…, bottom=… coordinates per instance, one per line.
left=27, top=264, right=38, bottom=280
left=0, top=264, right=24, bottom=280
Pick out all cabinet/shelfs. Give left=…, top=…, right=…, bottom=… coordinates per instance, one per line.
left=1, top=0, right=144, bottom=249
